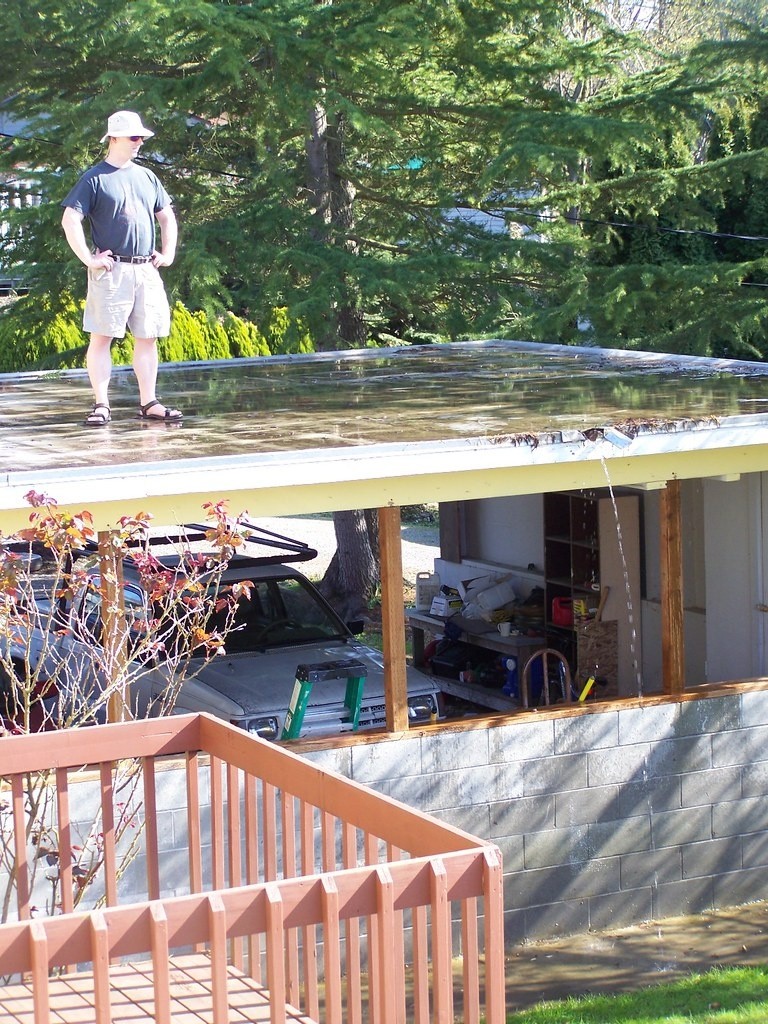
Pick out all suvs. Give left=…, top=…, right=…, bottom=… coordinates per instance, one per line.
left=0, top=516, right=448, bottom=744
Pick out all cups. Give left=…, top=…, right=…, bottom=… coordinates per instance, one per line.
left=497, top=622, right=510, bottom=637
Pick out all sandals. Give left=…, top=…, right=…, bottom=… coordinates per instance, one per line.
left=137, top=400, right=183, bottom=420
left=85, top=402, right=111, bottom=424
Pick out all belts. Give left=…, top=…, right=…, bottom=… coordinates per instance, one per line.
left=108, top=255, right=152, bottom=264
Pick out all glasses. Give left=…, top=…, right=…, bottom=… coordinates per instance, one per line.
left=130, top=136, right=144, bottom=142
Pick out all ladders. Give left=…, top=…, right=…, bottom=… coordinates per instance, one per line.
left=280, top=659, right=368, bottom=741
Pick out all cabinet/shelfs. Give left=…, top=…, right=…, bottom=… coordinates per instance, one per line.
left=539, top=490, right=641, bottom=700
left=401, top=607, right=549, bottom=713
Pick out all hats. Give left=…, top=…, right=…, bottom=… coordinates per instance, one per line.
left=100, top=111, right=154, bottom=143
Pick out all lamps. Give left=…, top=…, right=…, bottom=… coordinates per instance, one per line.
left=602, top=428, right=632, bottom=449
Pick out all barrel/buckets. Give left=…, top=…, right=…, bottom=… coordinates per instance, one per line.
left=415, top=572, right=440, bottom=611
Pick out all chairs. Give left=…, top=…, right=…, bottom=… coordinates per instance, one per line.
left=210, top=592, right=260, bottom=655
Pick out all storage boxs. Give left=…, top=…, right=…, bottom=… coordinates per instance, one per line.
left=430, top=595, right=462, bottom=616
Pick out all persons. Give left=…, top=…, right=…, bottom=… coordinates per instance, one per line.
left=60, top=109, right=183, bottom=425
